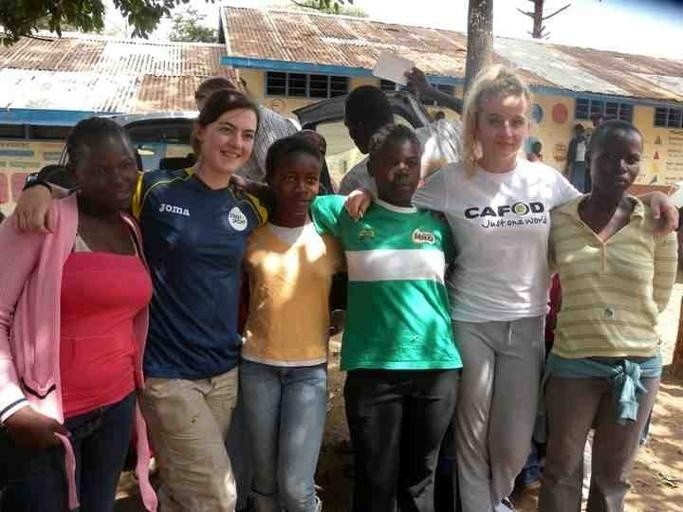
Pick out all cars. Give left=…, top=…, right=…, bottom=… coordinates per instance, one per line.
left=59, top=90, right=434, bottom=195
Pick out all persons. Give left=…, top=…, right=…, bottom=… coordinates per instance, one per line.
left=0, top=65, right=682, bottom=511
